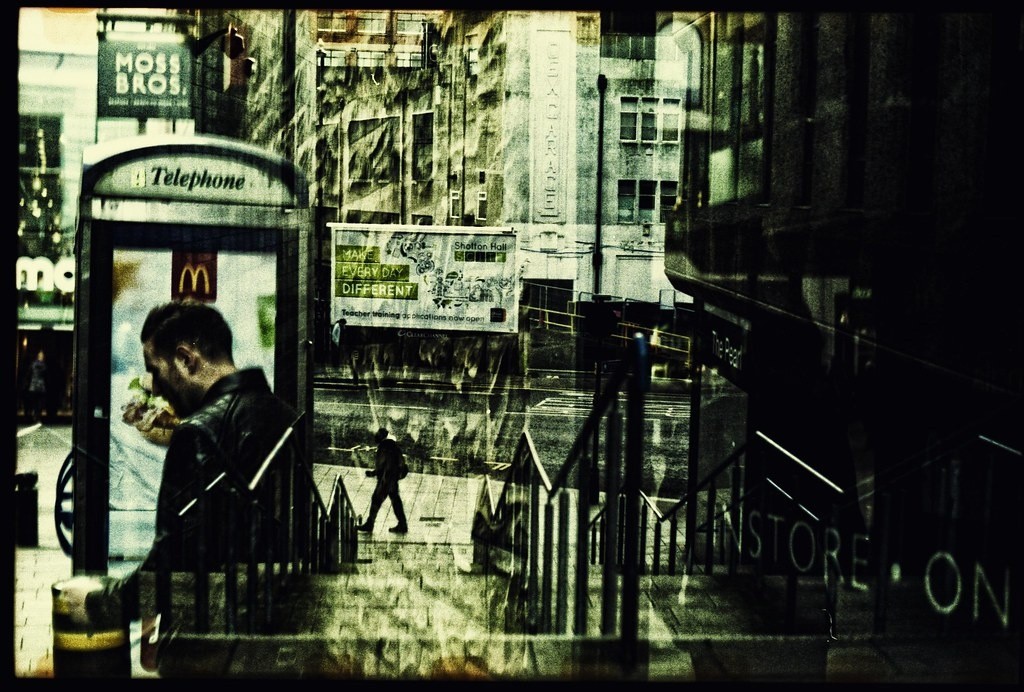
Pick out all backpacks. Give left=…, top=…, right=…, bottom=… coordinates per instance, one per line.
left=389, top=439, right=408, bottom=481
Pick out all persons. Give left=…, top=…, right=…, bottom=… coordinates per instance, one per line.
left=61, top=298, right=313, bottom=679
left=22, top=351, right=55, bottom=425
left=355, top=428, right=408, bottom=534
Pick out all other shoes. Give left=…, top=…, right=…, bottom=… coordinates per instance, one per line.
left=356, top=522, right=373, bottom=532
left=389, top=522, right=407, bottom=533
